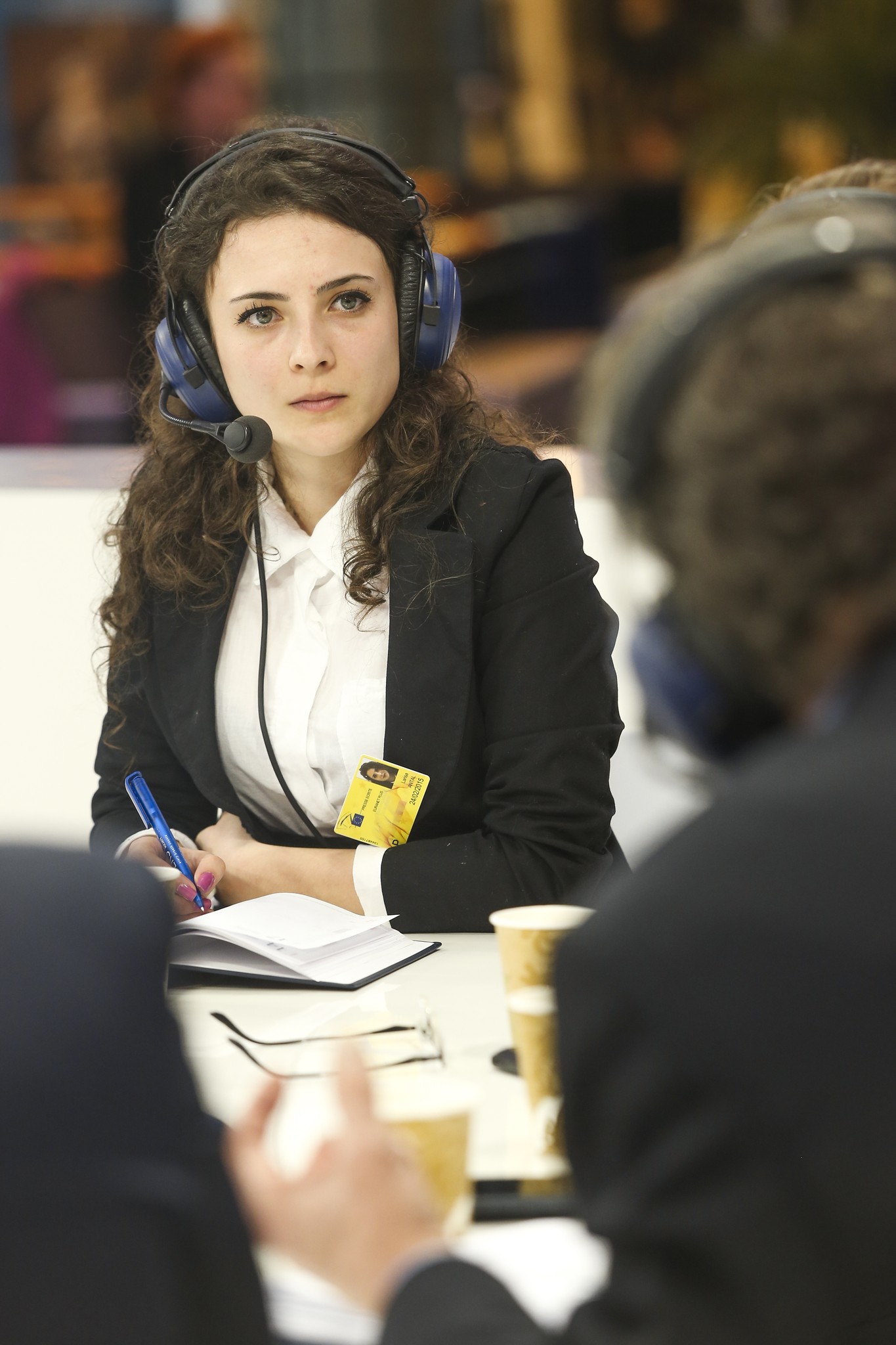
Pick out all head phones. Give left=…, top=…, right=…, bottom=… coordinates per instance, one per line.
left=587, top=192, right=896, bottom=764
left=155, top=129, right=463, bottom=422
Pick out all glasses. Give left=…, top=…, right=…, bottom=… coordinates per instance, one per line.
left=212, top=1012, right=448, bottom=1078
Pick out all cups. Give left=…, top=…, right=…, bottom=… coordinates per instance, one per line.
left=509, top=986, right=561, bottom=1106
left=380, top=1091, right=472, bottom=1236
left=488, top=903, right=596, bottom=989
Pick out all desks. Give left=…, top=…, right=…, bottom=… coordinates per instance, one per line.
left=166, top=932, right=612, bottom=1345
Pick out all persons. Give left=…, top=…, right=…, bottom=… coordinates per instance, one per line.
left=3, top=159, right=896, bottom=1345
left=86, top=115, right=643, bottom=930
left=359, top=761, right=399, bottom=789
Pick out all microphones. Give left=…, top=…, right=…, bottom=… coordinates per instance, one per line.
left=156, top=373, right=271, bottom=464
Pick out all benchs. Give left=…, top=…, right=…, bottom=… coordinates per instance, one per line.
left=1, top=439, right=713, bottom=859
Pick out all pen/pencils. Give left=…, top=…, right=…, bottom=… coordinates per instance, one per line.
left=124, top=772, right=205, bottom=913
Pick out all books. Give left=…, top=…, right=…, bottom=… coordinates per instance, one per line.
left=166, top=891, right=443, bottom=992
